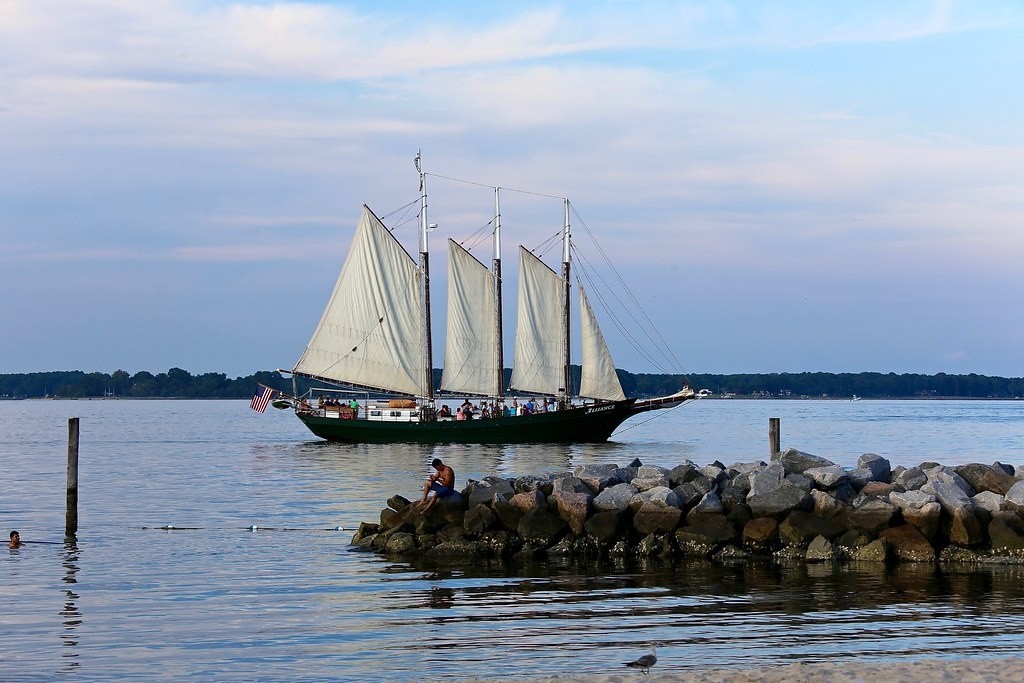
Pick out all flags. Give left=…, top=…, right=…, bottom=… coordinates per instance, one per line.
left=248, top=382, right=274, bottom=413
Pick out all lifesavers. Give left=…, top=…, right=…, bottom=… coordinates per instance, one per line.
left=341, top=409, right=352, bottom=419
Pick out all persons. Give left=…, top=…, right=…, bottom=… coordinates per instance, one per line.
left=436, top=391, right=602, bottom=421
left=415, top=458, right=455, bottom=515
left=293, top=392, right=364, bottom=420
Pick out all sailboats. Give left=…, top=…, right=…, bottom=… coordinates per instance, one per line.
left=271, top=153, right=696, bottom=442
